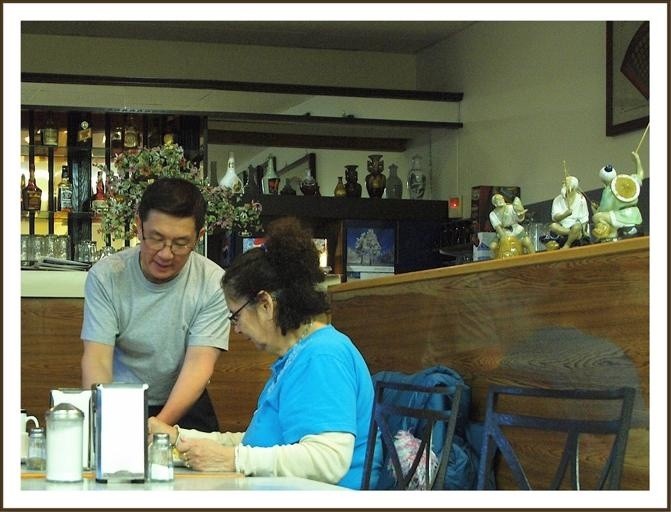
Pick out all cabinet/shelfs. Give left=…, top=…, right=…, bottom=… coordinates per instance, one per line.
left=222, top=198, right=447, bottom=283
left=21, top=108, right=208, bottom=271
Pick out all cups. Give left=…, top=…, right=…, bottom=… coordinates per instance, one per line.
left=521, top=219, right=596, bottom=252
left=21, top=408, right=40, bottom=465
left=21, top=233, right=133, bottom=264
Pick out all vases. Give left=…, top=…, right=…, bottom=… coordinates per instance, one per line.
left=219, top=148, right=428, bottom=200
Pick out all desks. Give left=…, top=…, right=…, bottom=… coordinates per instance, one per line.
left=19, top=453, right=357, bottom=493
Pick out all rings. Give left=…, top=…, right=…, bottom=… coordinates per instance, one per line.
left=185, top=461, right=190, bottom=469
left=183, top=452, right=188, bottom=461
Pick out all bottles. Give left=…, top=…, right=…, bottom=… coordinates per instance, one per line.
left=89, top=168, right=126, bottom=215
left=218, top=150, right=244, bottom=195
left=262, top=152, right=280, bottom=195
left=43, top=403, right=86, bottom=483
left=332, top=153, right=427, bottom=201
left=280, top=179, right=297, bottom=195
left=300, top=168, right=316, bottom=196
left=40, top=110, right=179, bottom=150
left=55, top=164, right=73, bottom=211
left=431, top=219, right=473, bottom=249
left=147, top=432, right=175, bottom=482
left=23, top=163, right=43, bottom=212
left=25, top=426, right=46, bottom=471
left=243, top=165, right=259, bottom=198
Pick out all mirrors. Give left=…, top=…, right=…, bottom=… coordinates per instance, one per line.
left=196, top=137, right=437, bottom=200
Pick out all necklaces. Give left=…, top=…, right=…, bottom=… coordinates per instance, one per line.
left=296, top=319, right=313, bottom=344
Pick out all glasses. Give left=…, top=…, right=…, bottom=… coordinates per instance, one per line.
left=228, top=300, right=251, bottom=324
left=141, top=216, right=200, bottom=257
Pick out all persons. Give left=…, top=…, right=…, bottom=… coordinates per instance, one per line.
left=489, top=194, right=536, bottom=259
left=550, top=175, right=589, bottom=249
left=81, top=177, right=230, bottom=431
left=592, top=151, right=644, bottom=239
left=148, top=215, right=384, bottom=491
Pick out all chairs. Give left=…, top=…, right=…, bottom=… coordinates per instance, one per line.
left=359, top=379, right=464, bottom=492
left=474, top=384, right=635, bottom=491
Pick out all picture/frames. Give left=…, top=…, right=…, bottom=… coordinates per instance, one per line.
left=604, top=20, right=650, bottom=136
